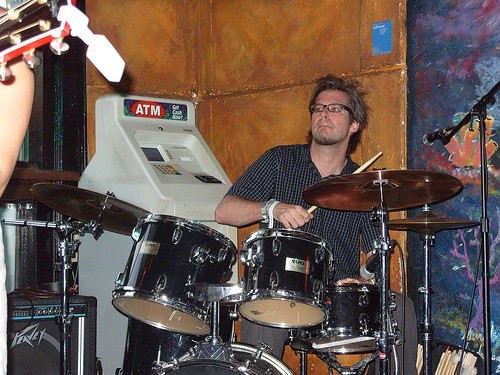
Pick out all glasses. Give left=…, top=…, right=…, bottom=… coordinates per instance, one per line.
left=309, top=103, right=356, bottom=119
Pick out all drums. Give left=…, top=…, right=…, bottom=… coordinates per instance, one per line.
left=290, top=281, right=389, bottom=356
left=146, top=341, right=298, bottom=375
left=110, top=212, right=237, bottom=338
left=238, top=227, right=335, bottom=330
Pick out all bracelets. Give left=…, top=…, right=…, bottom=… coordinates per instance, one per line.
left=261, top=197, right=279, bottom=229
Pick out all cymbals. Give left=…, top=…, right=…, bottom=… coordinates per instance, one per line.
left=375, top=212, right=482, bottom=234
left=0, top=162, right=80, bottom=204
left=28, top=180, right=153, bottom=238
left=300, top=169, right=465, bottom=213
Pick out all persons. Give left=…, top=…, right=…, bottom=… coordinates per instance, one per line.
left=0, top=0, right=35, bottom=375
left=215, top=73, right=388, bottom=287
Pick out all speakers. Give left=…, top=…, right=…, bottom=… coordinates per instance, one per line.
left=6, top=290, right=97, bottom=375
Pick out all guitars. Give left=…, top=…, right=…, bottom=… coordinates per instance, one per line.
left=0, top=0, right=127, bottom=84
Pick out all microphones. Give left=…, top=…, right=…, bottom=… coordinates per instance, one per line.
left=423, top=126, right=458, bottom=147
